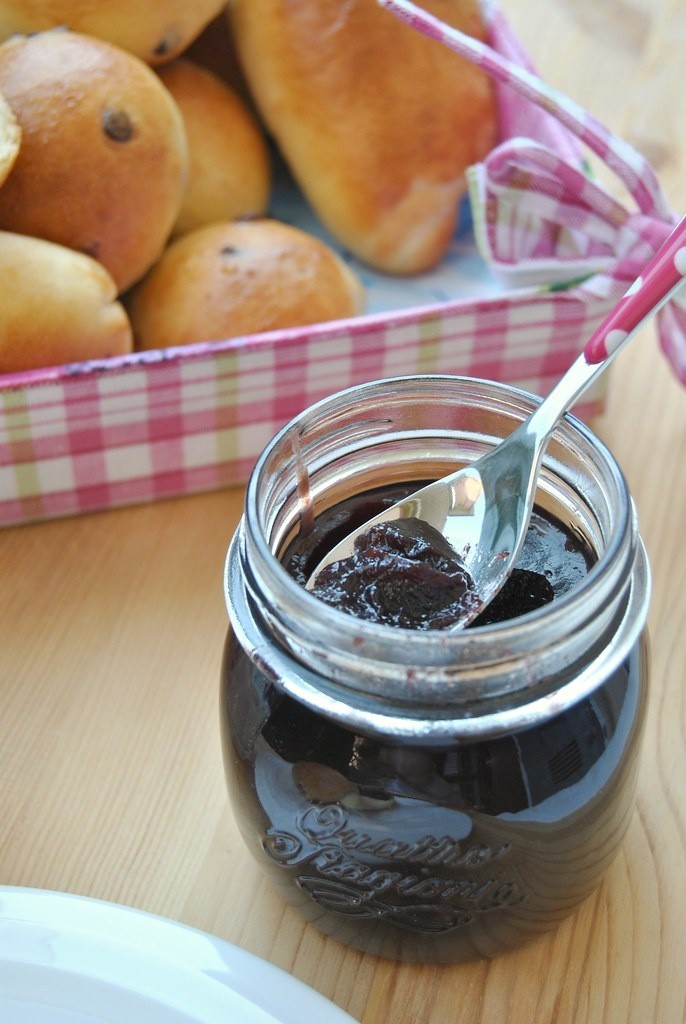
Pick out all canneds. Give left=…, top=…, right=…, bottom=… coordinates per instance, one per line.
left=222, top=377, right=653, bottom=966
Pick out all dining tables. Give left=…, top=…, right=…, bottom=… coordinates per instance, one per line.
left=0, top=0, right=686, bottom=1024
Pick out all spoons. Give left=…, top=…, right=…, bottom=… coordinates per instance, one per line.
left=306, top=214, right=686, bottom=631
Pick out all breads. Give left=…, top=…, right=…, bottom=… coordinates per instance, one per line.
left=225, top=1, right=504, bottom=282
left=3, top=0, right=361, bottom=374
